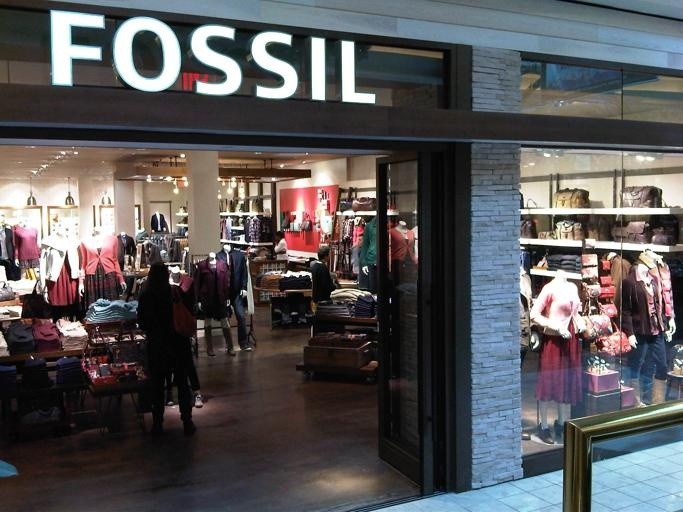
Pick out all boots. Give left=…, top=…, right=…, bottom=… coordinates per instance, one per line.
left=204, top=326, right=215, bottom=357
left=223, top=328, right=236, bottom=356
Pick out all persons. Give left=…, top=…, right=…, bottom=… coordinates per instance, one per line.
left=215, top=244, right=253, bottom=352
left=0, top=223, right=15, bottom=279
left=530, top=268, right=588, bottom=443
left=636, top=250, right=676, bottom=401
left=195, top=252, right=237, bottom=357
left=273, top=231, right=289, bottom=273
left=39, top=228, right=80, bottom=323
left=166, top=266, right=203, bottom=407
left=622, top=264, right=668, bottom=407
left=150, top=211, right=168, bottom=232
left=310, top=246, right=338, bottom=305
left=519, top=266, right=540, bottom=439
left=359, top=216, right=377, bottom=294
left=115, top=232, right=136, bottom=295
left=388, top=221, right=418, bottom=291
left=11, top=217, right=42, bottom=271
left=78, top=227, right=128, bottom=318
left=137, top=261, right=196, bottom=435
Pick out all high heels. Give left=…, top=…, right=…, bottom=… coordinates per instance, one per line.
left=554, top=420, right=564, bottom=436
left=537, top=422, right=553, bottom=444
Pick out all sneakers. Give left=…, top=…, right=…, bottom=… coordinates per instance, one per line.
left=194, top=393, right=203, bottom=408
left=183, top=419, right=196, bottom=434
left=149, top=425, right=163, bottom=433
left=240, top=344, right=253, bottom=351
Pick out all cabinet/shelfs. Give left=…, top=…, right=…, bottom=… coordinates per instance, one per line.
left=294, top=310, right=377, bottom=382
left=174, top=210, right=273, bottom=248
left=519, top=203, right=682, bottom=282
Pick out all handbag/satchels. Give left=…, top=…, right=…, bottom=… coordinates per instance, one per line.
left=520, top=185, right=677, bottom=245
left=173, top=285, right=198, bottom=338
left=339, top=195, right=376, bottom=211
left=18, top=403, right=66, bottom=439
left=580, top=244, right=632, bottom=356
